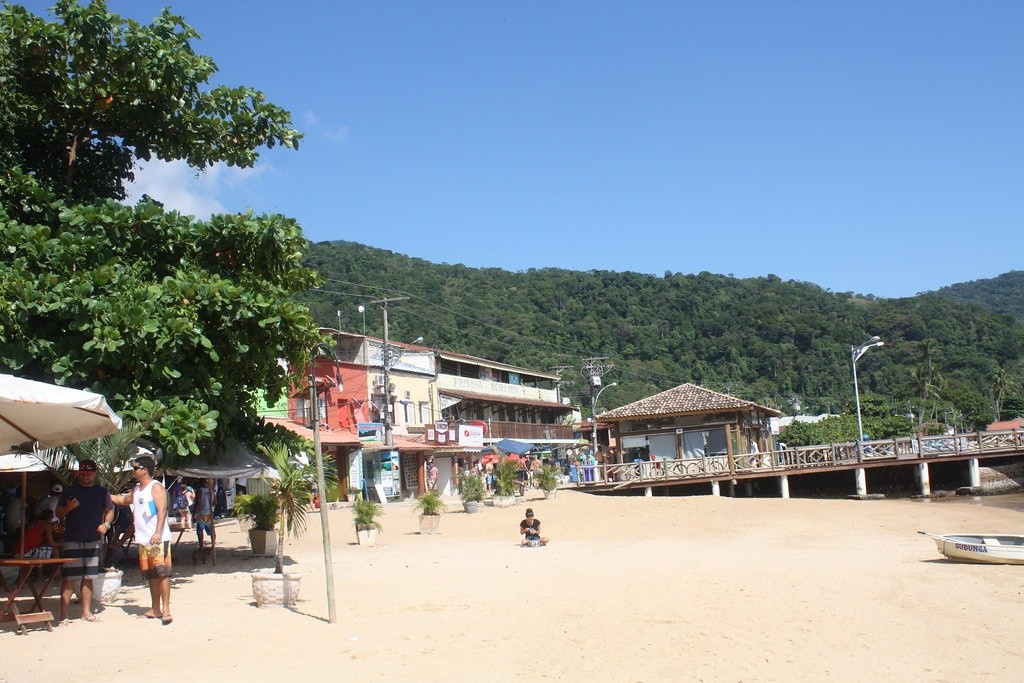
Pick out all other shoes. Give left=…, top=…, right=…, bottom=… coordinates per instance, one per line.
left=40, top=578, right=50, bottom=584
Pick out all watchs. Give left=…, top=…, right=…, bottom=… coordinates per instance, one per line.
left=105, top=523, right=111, bottom=529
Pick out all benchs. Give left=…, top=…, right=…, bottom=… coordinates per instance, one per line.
left=983, top=538, right=1001, bottom=546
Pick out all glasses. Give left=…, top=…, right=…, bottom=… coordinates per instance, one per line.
left=134, top=465, right=144, bottom=470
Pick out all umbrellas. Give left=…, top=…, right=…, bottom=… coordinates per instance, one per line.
left=0, top=374, right=121, bottom=455
left=481, top=438, right=535, bottom=455
left=0, top=446, right=80, bottom=557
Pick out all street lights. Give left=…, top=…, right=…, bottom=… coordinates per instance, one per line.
left=358, top=303, right=366, bottom=366
left=851, top=336, right=885, bottom=442
left=383, top=336, right=424, bottom=446
left=590, top=382, right=617, bottom=466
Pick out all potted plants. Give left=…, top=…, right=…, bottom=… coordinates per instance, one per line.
left=451, top=466, right=485, bottom=513
left=352, top=500, right=382, bottom=547
left=487, top=443, right=522, bottom=508
left=253, top=442, right=338, bottom=608
left=414, top=488, right=447, bottom=535
left=7, top=414, right=154, bottom=605
left=345, top=486, right=361, bottom=502
left=532, top=462, right=561, bottom=500
left=234, top=494, right=279, bottom=555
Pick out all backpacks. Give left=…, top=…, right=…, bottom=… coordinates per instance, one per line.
left=177, top=491, right=189, bottom=508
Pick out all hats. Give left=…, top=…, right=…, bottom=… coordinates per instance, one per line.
left=78, top=460, right=97, bottom=470
left=203, top=480, right=209, bottom=487
left=187, top=486, right=193, bottom=491
left=40, top=509, right=54, bottom=517
left=51, top=484, right=63, bottom=492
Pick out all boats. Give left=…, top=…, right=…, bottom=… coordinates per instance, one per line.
left=917, top=531, right=1024, bottom=564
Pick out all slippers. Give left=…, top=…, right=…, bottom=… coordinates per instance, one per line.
left=162, top=615, right=173, bottom=622
left=141, top=613, right=162, bottom=618
left=82, top=614, right=99, bottom=622
left=57, top=620, right=69, bottom=627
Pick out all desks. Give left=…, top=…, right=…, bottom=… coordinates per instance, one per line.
left=0, top=559, right=79, bottom=635
left=116, top=525, right=190, bottom=562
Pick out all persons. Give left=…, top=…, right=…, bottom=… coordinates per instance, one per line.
left=6, top=483, right=67, bottom=578
left=776, top=442, right=784, bottom=462
left=572, top=451, right=595, bottom=467
left=750, top=439, right=759, bottom=467
left=179, top=485, right=195, bottom=530
left=428, top=462, right=438, bottom=490
left=55, top=459, right=115, bottom=626
left=519, top=508, right=549, bottom=548
left=110, top=457, right=173, bottom=624
left=194, top=478, right=216, bottom=548
left=470, top=453, right=541, bottom=492
